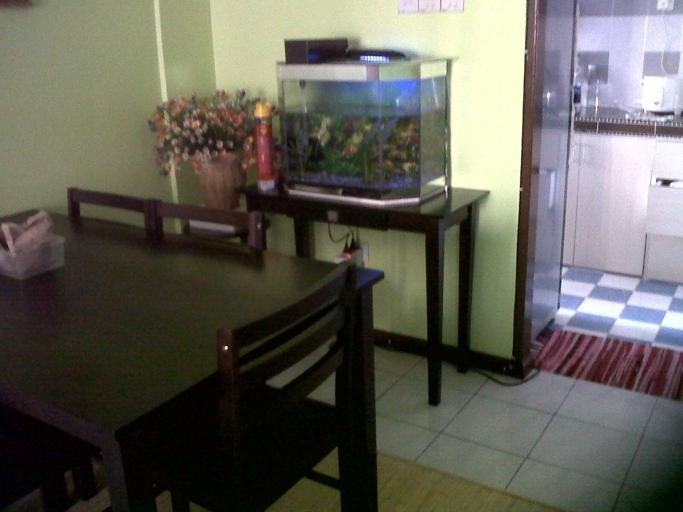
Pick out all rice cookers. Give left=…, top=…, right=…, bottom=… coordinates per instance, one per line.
left=641, top=74, right=681, bottom=114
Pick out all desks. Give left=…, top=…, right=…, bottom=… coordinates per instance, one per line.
left=235, top=182, right=490, bottom=405
left=0, top=210, right=384, bottom=512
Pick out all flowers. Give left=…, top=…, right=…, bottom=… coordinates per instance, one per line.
left=148, top=90, right=282, bottom=177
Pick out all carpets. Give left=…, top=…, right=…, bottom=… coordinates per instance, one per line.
left=531, top=329, right=682, bottom=400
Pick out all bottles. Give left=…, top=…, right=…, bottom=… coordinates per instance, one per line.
left=253, top=100, right=275, bottom=192
left=573, top=62, right=600, bottom=114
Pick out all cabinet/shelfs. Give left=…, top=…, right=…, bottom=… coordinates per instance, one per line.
left=563, top=132, right=682, bottom=284
